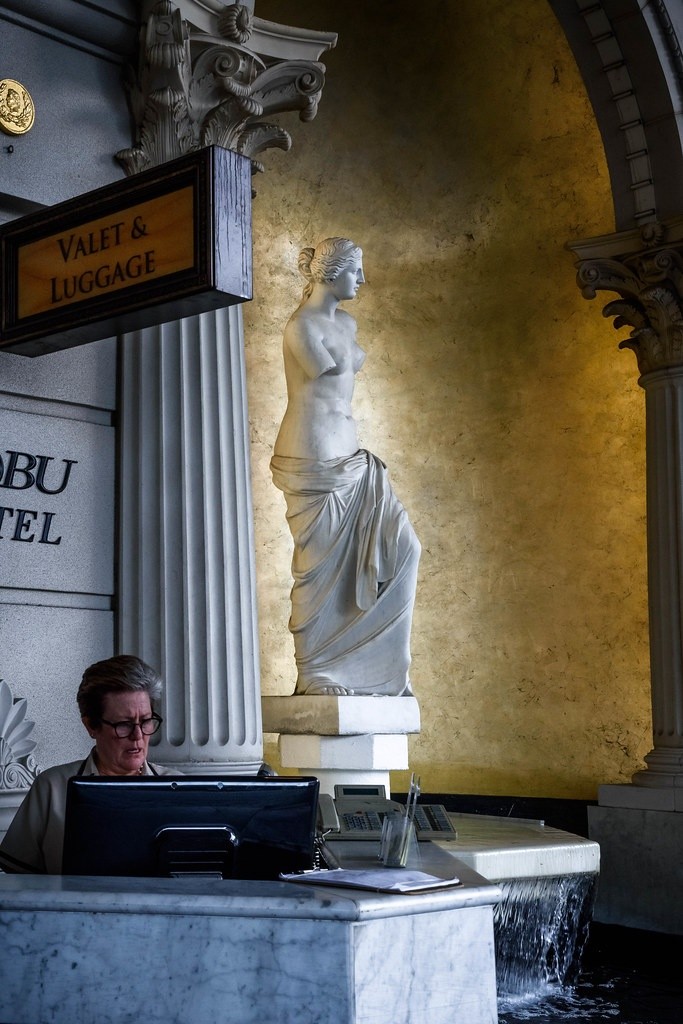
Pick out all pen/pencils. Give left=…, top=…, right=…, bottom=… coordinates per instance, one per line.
left=291, top=868, right=328, bottom=874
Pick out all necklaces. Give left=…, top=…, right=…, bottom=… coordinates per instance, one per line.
left=135, top=765, right=145, bottom=775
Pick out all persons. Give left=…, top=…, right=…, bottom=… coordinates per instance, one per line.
left=0, top=652, right=184, bottom=891
left=266, top=235, right=425, bottom=701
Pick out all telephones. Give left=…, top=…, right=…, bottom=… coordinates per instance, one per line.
left=317, top=785, right=405, bottom=842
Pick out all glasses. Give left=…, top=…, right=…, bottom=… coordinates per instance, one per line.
left=102, top=711, right=163, bottom=738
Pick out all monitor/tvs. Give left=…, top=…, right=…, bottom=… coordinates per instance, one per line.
left=62, top=776, right=319, bottom=881
